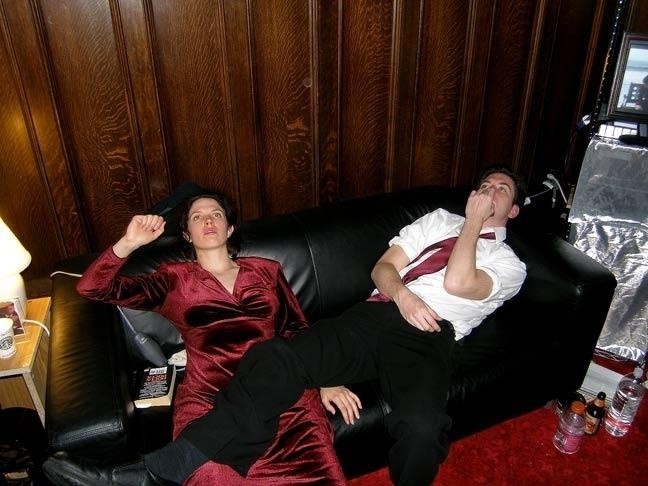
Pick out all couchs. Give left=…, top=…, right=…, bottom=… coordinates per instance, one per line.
left=49, top=186, right=617, bottom=485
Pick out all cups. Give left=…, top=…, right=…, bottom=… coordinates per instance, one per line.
left=0, top=317, right=17, bottom=360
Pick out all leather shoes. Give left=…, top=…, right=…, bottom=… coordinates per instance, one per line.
left=40, top=453, right=169, bottom=486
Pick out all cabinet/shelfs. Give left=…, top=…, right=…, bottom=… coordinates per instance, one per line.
left=1, top=0, right=629, bottom=270
left=0, top=297, right=52, bottom=433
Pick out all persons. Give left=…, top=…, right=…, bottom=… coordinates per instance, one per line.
left=76, top=186, right=366, bottom=485
left=41, top=166, right=529, bottom=485
left=2, top=301, right=20, bottom=334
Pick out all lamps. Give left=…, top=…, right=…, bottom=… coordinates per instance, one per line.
left=0, top=217, right=33, bottom=339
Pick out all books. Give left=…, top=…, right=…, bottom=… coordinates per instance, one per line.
left=132, top=363, right=177, bottom=409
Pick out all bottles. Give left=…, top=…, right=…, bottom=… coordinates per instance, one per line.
left=551, top=401, right=587, bottom=456
left=585, top=391, right=606, bottom=437
left=603, top=367, right=646, bottom=438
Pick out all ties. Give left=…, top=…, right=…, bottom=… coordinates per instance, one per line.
left=367, top=232, right=494, bottom=302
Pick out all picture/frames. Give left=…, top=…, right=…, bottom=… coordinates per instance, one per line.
left=607, top=32, right=648, bottom=124
